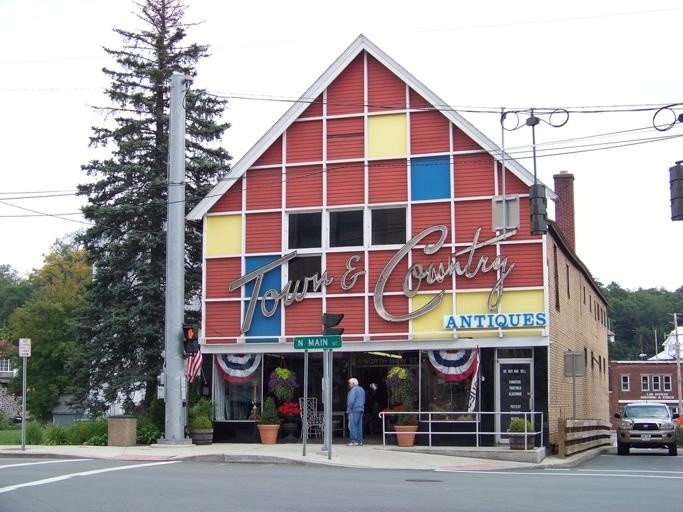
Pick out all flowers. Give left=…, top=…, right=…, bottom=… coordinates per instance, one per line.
left=385, top=366, right=414, bottom=403
left=269, top=366, right=300, bottom=401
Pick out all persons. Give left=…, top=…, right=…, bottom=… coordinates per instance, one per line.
left=346, top=377, right=366, bottom=446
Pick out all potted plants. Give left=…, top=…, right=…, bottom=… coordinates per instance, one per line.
left=508, top=418, right=537, bottom=449
left=190, top=414, right=214, bottom=445
left=393, top=399, right=419, bottom=447
left=256, top=396, right=281, bottom=444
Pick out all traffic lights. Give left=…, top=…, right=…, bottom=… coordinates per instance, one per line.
left=182, top=324, right=200, bottom=356
left=323, top=313, right=344, bottom=348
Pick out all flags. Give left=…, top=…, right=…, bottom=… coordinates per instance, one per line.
left=188, top=350, right=203, bottom=383
left=468, top=348, right=481, bottom=412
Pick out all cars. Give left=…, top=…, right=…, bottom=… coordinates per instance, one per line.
left=615, top=403, right=679, bottom=456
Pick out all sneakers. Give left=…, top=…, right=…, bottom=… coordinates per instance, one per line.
left=347, top=442, right=363, bottom=446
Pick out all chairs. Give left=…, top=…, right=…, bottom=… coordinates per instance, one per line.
left=298, top=397, right=324, bottom=441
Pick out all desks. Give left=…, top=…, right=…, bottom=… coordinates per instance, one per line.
left=318, top=410, right=347, bottom=440
